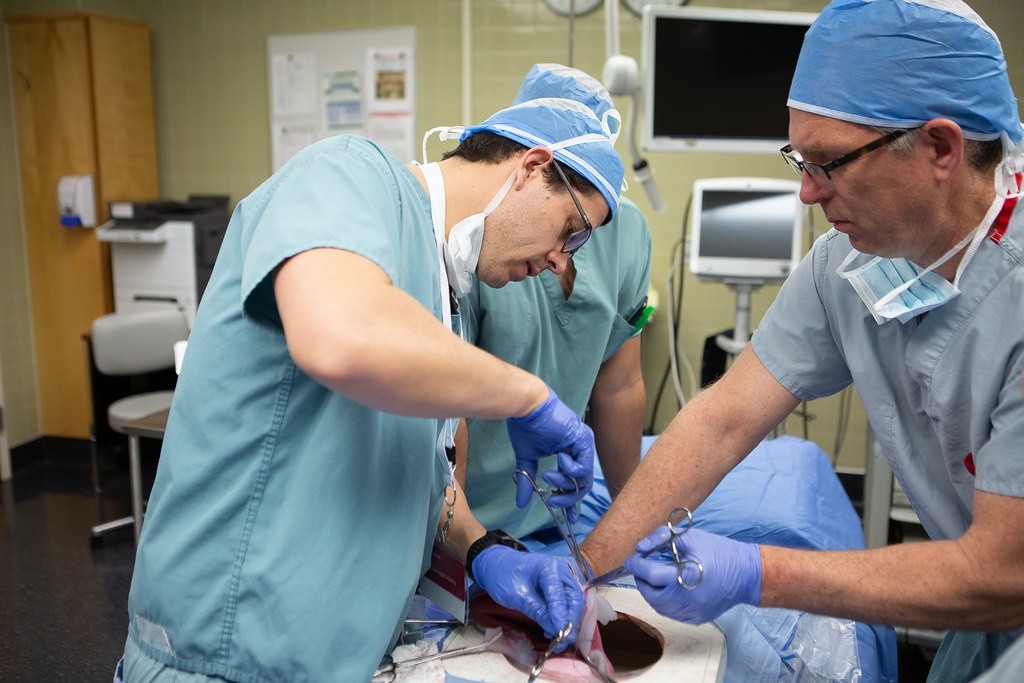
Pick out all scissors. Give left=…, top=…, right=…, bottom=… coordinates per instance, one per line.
left=372, top=617, right=575, bottom=683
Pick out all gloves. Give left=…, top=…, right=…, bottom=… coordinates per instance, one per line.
left=555, top=556, right=595, bottom=586
left=471, top=545, right=586, bottom=653
left=623, top=525, right=763, bottom=626
left=507, top=386, right=595, bottom=523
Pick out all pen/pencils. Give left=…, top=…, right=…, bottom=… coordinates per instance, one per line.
left=622, top=295, right=657, bottom=337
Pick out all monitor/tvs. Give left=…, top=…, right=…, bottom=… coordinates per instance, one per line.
left=641, top=4, right=821, bottom=153
left=689, top=176, right=804, bottom=280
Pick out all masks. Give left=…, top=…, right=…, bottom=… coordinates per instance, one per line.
left=444, top=167, right=516, bottom=299
left=836, top=197, right=1006, bottom=326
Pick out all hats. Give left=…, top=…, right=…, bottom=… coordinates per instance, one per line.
left=423, top=98, right=624, bottom=227
left=787, top=1, right=1024, bottom=199
left=512, top=63, right=620, bottom=146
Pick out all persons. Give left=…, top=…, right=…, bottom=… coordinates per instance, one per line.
left=567, top=0, right=1024, bottom=683
left=457, top=63, right=652, bottom=541
left=113, top=98, right=624, bottom=683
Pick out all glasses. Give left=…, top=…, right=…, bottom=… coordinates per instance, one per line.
left=780, top=129, right=915, bottom=182
left=553, top=159, right=592, bottom=260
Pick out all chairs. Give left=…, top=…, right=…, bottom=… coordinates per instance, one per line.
left=91, top=306, right=189, bottom=545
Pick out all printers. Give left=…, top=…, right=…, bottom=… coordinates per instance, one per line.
left=96, top=192, right=230, bottom=332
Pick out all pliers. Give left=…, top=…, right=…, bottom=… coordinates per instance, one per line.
left=584, top=506, right=704, bottom=592
left=511, top=468, right=592, bottom=584
